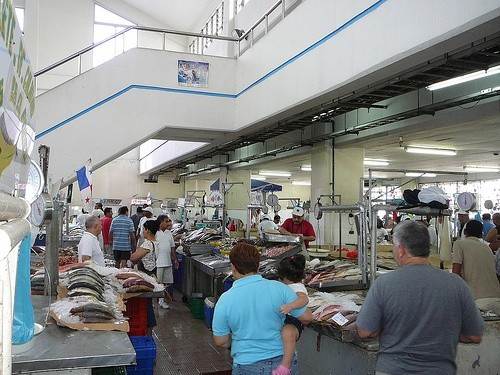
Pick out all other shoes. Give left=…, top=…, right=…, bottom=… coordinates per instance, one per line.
left=272, top=364, right=292, bottom=375
left=158, top=302, right=169, bottom=309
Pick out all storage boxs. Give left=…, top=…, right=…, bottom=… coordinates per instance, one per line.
left=126, top=336, right=157, bottom=375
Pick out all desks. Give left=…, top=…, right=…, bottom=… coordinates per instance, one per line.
left=190, top=255, right=231, bottom=298
left=9, top=294, right=137, bottom=375
left=123, top=288, right=166, bottom=299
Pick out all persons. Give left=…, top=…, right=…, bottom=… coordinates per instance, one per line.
left=130, top=220, right=161, bottom=330
left=279, top=206, right=316, bottom=251
left=91, top=203, right=104, bottom=219
left=212, top=242, right=313, bottom=375
left=367, top=209, right=496, bottom=252
left=272, top=254, right=309, bottom=375
left=259, top=214, right=281, bottom=238
left=356, top=220, right=485, bottom=375
left=136, top=206, right=156, bottom=237
left=452, top=220, right=500, bottom=316
left=78, top=216, right=106, bottom=269
left=484, top=213, right=500, bottom=251
left=166, top=219, right=182, bottom=301
left=129, top=203, right=149, bottom=242
left=108, top=206, right=137, bottom=269
left=156, top=215, right=179, bottom=309
left=100, top=208, right=113, bottom=252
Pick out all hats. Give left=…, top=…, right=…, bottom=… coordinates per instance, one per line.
left=142, top=207, right=153, bottom=214
left=292, top=207, right=304, bottom=216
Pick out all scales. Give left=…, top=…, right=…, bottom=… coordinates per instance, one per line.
left=267, top=194, right=278, bottom=211
left=29, top=195, right=48, bottom=227
left=306, top=198, right=330, bottom=257
left=457, top=191, right=476, bottom=213
left=274, top=204, right=281, bottom=215
left=262, top=206, right=268, bottom=215
left=484, top=200, right=493, bottom=210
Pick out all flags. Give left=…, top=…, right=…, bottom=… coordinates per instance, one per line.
left=74, top=159, right=93, bottom=213
left=210, top=178, right=224, bottom=208
left=147, top=193, right=152, bottom=205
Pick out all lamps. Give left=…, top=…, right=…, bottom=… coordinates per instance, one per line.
left=251, top=167, right=311, bottom=184
left=231, top=29, right=248, bottom=43
left=399, top=142, right=457, bottom=155
left=425, top=65, right=500, bottom=90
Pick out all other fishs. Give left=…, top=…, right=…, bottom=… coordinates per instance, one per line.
left=66, top=265, right=110, bottom=301
left=68, top=303, right=120, bottom=324
left=315, top=304, right=359, bottom=327
left=116, top=271, right=155, bottom=293
